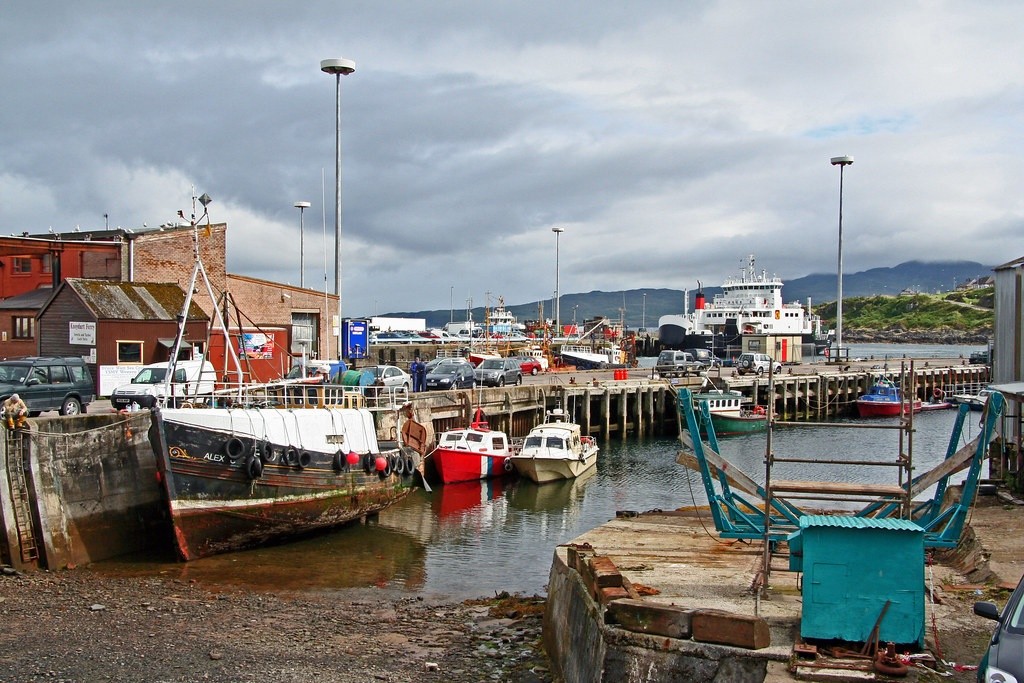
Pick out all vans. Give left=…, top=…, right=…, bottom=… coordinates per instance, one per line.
left=276, top=359, right=348, bottom=405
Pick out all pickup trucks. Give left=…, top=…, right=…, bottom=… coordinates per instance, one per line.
left=111, top=361, right=217, bottom=410
left=656, top=348, right=721, bottom=377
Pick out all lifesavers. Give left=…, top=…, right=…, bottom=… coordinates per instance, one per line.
left=334, top=451, right=348, bottom=471
left=247, top=440, right=275, bottom=480
left=364, top=453, right=376, bottom=473
left=381, top=460, right=391, bottom=477
left=283, top=445, right=312, bottom=468
left=404, top=456, right=415, bottom=473
left=932, top=388, right=942, bottom=398
left=226, top=437, right=246, bottom=458
left=396, top=457, right=405, bottom=474
left=388, top=455, right=397, bottom=472
left=475, top=410, right=487, bottom=428
left=753, top=406, right=764, bottom=415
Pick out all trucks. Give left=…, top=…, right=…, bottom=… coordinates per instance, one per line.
left=364, top=316, right=475, bottom=337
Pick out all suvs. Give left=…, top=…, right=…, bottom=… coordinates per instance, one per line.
left=0, top=356, right=96, bottom=416
left=737, top=352, right=782, bottom=375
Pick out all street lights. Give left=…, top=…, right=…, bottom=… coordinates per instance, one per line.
left=552, top=225, right=564, bottom=337
left=294, top=201, right=312, bottom=289
left=320, top=56, right=356, bottom=359
left=450, top=285, right=454, bottom=323
left=643, top=292, right=648, bottom=330
left=830, top=156, right=855, bottom=363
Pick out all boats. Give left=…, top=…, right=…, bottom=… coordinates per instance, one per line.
left=431, top=358, right=522, bottom=484
left=510, top=404, right=600, bottom=484
left=675, top=366, right=778, bottom=436
left=146, top=186, right=422, bottom=559
left=479, top=296, right=657, bottom=371
left=855, top=375, right=952, bottom=417
left=952, top=394, right=988, bottom=411
left=658, top=254, right=835, bottom=361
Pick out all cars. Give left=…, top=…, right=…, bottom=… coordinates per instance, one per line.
left=976, top=573, right=1024, bottom=683
left=424, top=357, right=478, bottom=391
left=516, top=356, right=542, bottom=376
left=474, top=357, right=522, bottom=388
left=359, top=365, right=413, bottom=394
left=369, top=329, right=527, bottom=344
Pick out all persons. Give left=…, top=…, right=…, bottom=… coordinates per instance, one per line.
left=411, top=356, right=421, bottom=392
left=417, top=358, right=427, bottom=392
left=5, top=393, right=29, bottom=430
